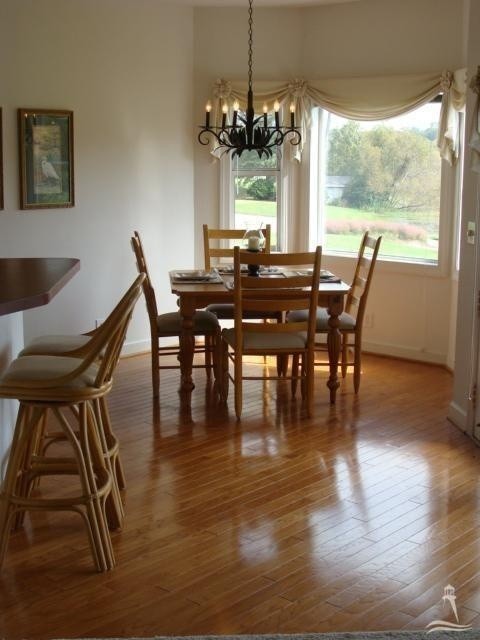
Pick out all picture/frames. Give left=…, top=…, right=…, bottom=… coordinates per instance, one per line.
left=0, top=107, right=75, bottom=211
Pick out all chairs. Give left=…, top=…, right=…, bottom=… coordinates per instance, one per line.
left=129, top=225, right=383, bottom=425
left=1, top=273, right=148, bottom=575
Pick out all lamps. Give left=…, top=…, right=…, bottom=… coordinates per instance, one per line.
left=197, top=0, right=304, bottom=159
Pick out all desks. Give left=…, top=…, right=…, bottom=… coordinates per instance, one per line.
left=0, top=258, right=82, bottom=497
